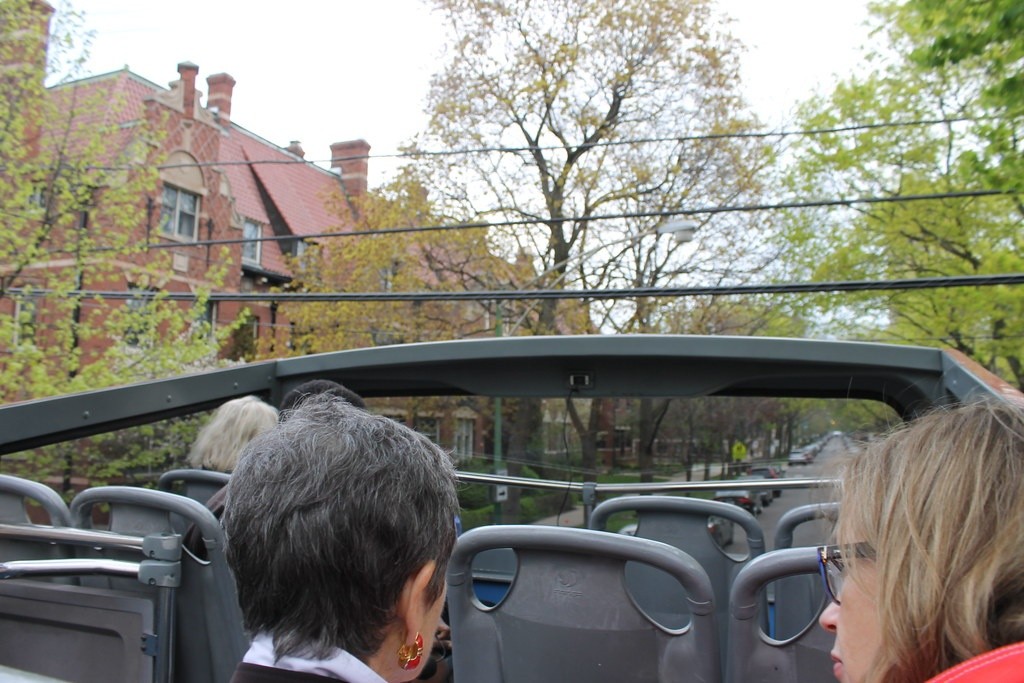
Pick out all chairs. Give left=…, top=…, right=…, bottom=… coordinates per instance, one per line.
left=587, top=496, right=770, bottom=683
left=726, top=546, right=843, bottom=683
left=0, top=521, right=182, bottom=683
left=157, top=469, right=233, bottom=537
left=444, top=526, right=719, bottom=683
left=774, top=500, right=844, bottom=642
left=70, top=486, right=250, bottom=683
left=0, top=475, right=79, bottom=585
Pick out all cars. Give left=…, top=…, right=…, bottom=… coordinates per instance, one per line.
left=708, top=513, right=733, bottom=548
left=739, top=473, right=774, bottom=505
left=715, top=488, right=759, bottom=515
left=745, top=464, right=783, bottom=496
left=788, top=433, right=834, bottom=465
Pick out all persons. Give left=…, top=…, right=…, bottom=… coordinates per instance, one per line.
left=819, top=395, right=1024, bottom=683
left=184, top=379, right=461, bottom=683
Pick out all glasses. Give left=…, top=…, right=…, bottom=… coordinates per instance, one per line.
left=817, top=541, right=876, bottom=607
